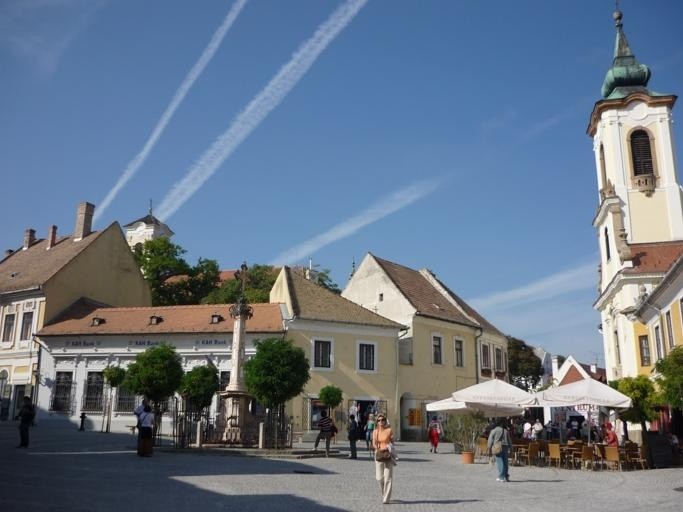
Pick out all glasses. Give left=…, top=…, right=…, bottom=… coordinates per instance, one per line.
left=377, top=419, right=385, bottom=422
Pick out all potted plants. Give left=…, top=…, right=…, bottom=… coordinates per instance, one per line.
left=444, top=409, right=489, bottom=464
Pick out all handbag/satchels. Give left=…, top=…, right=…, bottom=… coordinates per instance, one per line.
left=375, top=447, right=392, bottom=462
left=490, top=439, right=503, bottom=454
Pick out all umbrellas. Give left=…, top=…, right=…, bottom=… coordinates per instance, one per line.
left=426, top=396, right=525, bottom=423
left=534, top=378, right=633, bottom=443
left=452, top=379, right=537, bottom=423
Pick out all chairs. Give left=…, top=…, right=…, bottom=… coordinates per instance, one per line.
left=477, top=437, right=650, bottom=472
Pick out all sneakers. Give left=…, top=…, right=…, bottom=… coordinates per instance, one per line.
left=496, top=476, right=509, bottom=483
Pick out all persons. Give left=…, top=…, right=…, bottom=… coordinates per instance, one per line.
left=347, top=415, right=357, bottom=459
left=137, top=405, right=156, bottom=457
left=372, top=414, right=394, bottom=504
left=487, top=420, right=512, bottom=482
left=510, top=418, right=618, bottom=450
left=15, top=396, right=35, bottom=448
left=134, top=400, right=147, bottom=453
left=364, top=414, right=375, bottom=450
left=312, top=411, right=331, bottom=457
left=427, top=416, right=441, bottom=453
